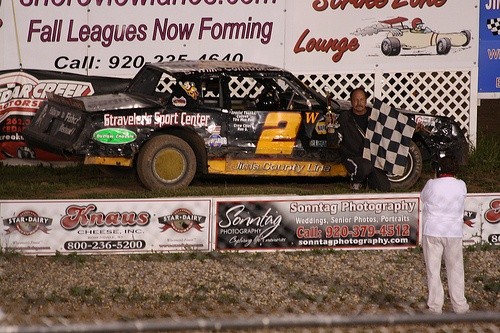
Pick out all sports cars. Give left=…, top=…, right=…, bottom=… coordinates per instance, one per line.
left=21, top=60, right=467, bottom=193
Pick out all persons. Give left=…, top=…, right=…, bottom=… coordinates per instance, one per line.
left=420, top=157, right=469, bottom=313
left=336, top=88, right=424, bottom=192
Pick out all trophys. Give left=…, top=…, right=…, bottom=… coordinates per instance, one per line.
left=324, top=93, right=338, bottom=134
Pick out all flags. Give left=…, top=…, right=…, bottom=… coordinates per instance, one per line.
left=362, top=97, right=418, bottom=177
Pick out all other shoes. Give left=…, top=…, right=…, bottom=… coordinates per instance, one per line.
left=348, top=181, right=363, bottom=191
